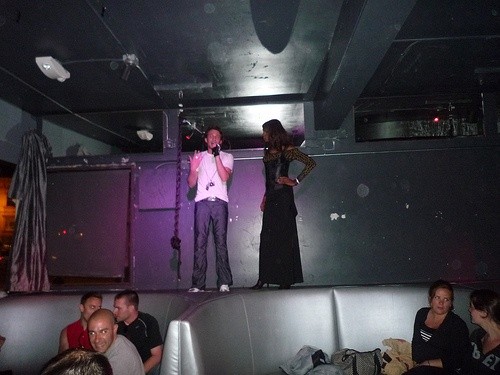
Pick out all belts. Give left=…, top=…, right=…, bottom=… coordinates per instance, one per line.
left=200, top=197, right=221, bottom=201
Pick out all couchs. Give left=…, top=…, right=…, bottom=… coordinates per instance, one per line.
left=182, top=286, right=481, bottom=375
left=0, top=294, right=188, bottom=375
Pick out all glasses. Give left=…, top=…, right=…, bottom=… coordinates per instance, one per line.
left=77, top=332, right=84, bottom=349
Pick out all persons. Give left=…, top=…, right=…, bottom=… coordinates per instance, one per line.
left=248, top=119, right=317, bottom=290
left=401, top=278, right=500, bottom=375
left=188, top=129, right=234, bottom=293
left=37, top=289, right=164, bottom=375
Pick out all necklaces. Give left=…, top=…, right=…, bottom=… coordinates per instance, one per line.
left=202, top=157, right=217, bottom=191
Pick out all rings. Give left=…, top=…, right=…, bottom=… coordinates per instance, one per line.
left=196, top=156, right=199, bottom=159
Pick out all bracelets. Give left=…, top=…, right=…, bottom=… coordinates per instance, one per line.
left=427, top=360, right=431, bottom=366
left=296, top=178, right=300, bottom=184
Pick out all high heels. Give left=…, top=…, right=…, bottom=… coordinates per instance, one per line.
left=248, top=279, right=269, bottom=290
left=280, top=284, right=291, bottom=289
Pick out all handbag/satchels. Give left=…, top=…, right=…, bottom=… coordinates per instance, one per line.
left=331, top=348, right=383, bottom=375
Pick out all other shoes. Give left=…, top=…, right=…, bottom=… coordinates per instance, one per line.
left=219, top=284, right=230, bottom=292
left=188, top=288, right=205, bottom=293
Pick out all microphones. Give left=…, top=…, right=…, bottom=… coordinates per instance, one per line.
left=212, top=144, right=216, bottom=148
